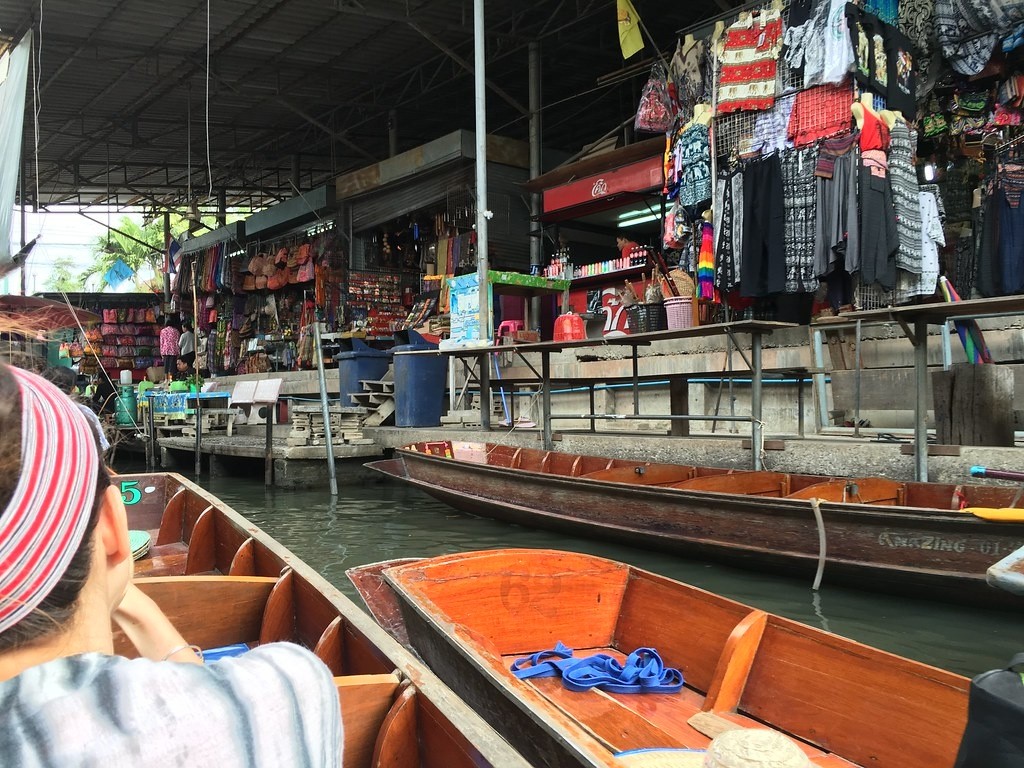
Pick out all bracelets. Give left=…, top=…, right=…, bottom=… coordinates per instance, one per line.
left=159, top=643, right=204, bottom=662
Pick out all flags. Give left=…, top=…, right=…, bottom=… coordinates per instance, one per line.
left=616, top=0, right=644, bottom=59
left=162, top=233, right=184, bottom=274
left=103, top=259, right=136, bottom=291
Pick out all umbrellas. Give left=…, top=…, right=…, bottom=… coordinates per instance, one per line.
left=695, top=220, right=720, bottom=323
left=0, top=293, right=101, bottom=371
left=940, top=276, right=995, bottom=364
left=618, top=264, right=664, bottom=305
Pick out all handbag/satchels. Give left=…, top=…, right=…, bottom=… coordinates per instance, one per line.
left=635, top=63, right=674, bottom=133
left=208, top=240, right=315, bottom=373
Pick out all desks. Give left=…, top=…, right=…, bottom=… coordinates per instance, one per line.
left=446, top=270, right=571, bottom=341
left=558, top=264, right=667, bottom=338
left=134, top=391, right=232, bottom=426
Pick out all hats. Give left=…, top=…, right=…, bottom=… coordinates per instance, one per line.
left=614, top=730, right=820, bottom=768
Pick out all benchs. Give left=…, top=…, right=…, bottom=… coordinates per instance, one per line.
left=186, top=408, right=241, bottom=436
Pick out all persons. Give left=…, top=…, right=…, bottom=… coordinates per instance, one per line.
left=160, top=319, right=200, bottom=383
left=850, top=92, right=921, bottom=292
left=616, top=234, right=644, bottom=257
left=674, top=11, right=748, bottom=209
left=0, top=359, right=344, bottom=768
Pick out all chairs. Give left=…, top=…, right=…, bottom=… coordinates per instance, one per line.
left=228, top=380, right=259, bottom=424
left=200, top=382, right=220, bottom=392
left=233, top=379, right=283, bottom=425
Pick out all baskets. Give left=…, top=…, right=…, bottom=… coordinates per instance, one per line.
left=625, top=304, right=663, bottom=333
left=664, top=296, right=692, bottom=329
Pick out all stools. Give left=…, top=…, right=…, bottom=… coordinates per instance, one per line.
left=493, top=320, right=524, bottom=356
left=102, top=414, right=116, bottom=425
left=553, top=315, right=586, bottom=341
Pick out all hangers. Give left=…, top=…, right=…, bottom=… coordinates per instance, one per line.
left=818, top=121, right=859, bottom=142
left=742, top=140, right=779, bottom=163
left=752, top=9, right=760, bottom=18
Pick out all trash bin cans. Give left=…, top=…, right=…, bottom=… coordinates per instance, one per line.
left=385, top=328, right=449, bottom=428
left=332, top=337, right=393, bottom=407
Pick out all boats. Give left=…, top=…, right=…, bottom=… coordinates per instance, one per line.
left=105, top=469, right=539, bottom=768
left=343, top=547, right=1024, bottom=768
left=362, top=439, right=1024, bottom=614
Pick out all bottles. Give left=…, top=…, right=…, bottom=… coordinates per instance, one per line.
left=544, top=244, right=648, bottom=279
